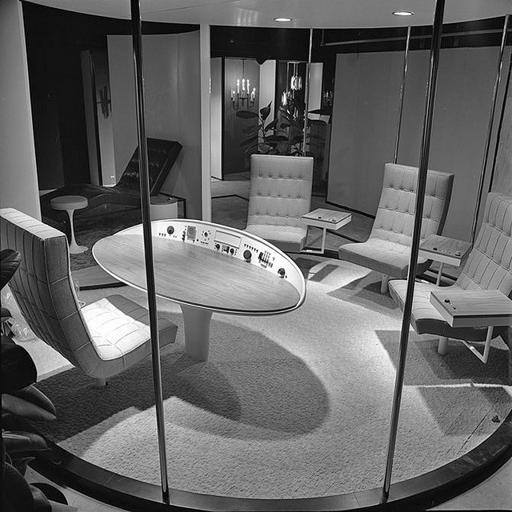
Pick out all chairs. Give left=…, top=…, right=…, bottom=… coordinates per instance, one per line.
left=242, top=153, right=314, bottom=255
left=1, top=209, right=178, bottom=391
left=390, top=191, right=512, bottom=358
left=336, top=161, right=455, bottom=296
left=39, top=134, right=182, bottom=222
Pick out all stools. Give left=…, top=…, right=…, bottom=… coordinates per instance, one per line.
left=50, top=193, right=93, bottom=256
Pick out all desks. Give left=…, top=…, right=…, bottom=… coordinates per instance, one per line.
left=415, top=233, right=474, bottom=289
left=430, top=288, right=512, bottom=364
left=91, top=219, right=305, bottom=363
left=300, top=206, right=354, bottom=255
